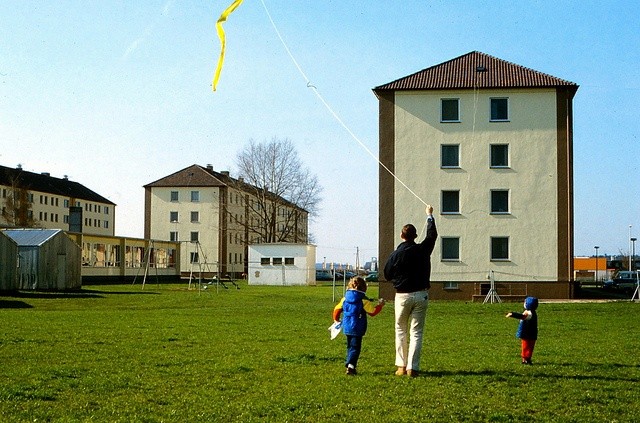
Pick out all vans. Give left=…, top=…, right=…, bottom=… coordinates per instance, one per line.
left=611, top=270, right=640, bottom=288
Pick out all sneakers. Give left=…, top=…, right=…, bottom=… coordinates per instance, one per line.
left=394, top=366, right=405, bottom=376
left=406, top=368, right=417, bottom=376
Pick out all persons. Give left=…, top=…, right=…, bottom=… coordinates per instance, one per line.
left=384, top=204, right=438, bottom=376
left=332, top=276, right=383, bottom=377
left=506, top=296, right=537, bottom=365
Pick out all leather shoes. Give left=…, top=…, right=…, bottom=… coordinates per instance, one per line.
left=347, top=367, right=358, bottom=375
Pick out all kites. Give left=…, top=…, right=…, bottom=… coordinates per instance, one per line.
left=211, top=0, right=245, bottom=92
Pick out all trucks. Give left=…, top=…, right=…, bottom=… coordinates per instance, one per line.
left=364, top=274, right=378, bottom=281
